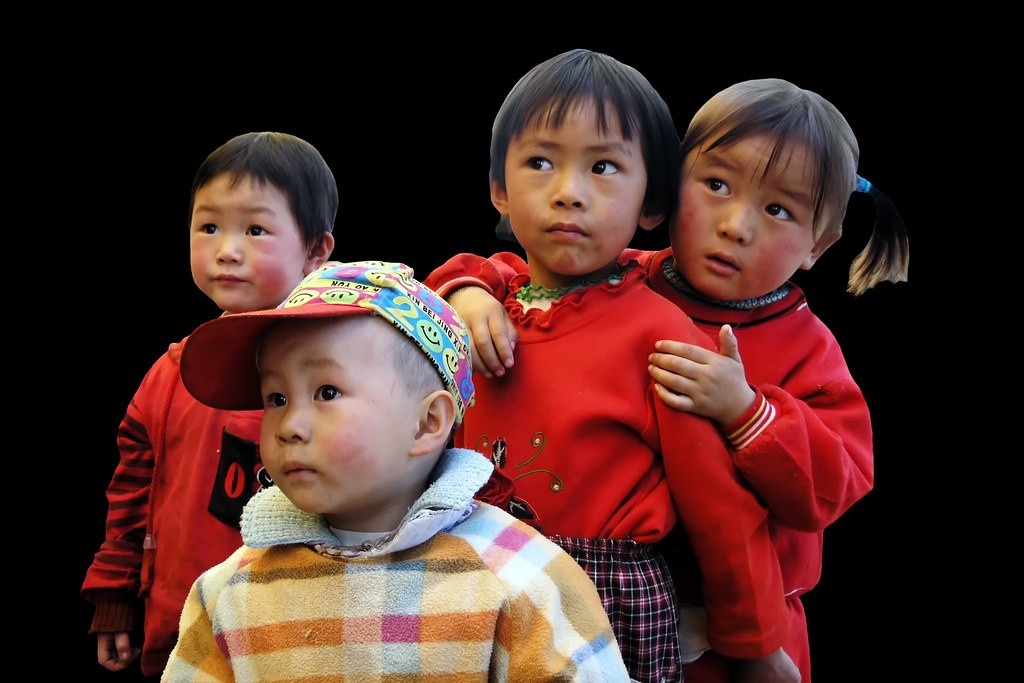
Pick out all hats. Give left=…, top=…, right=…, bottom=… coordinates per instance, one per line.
left=179, top=261, right=476, bottom=432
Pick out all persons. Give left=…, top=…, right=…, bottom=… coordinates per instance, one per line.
left=79, top=131, right=339, bottom=676
left=160, top=261, right=632, bottom=683
left=420, top=78, right=909, bottom=683
left=453, top=48, right=802, bottom=683
